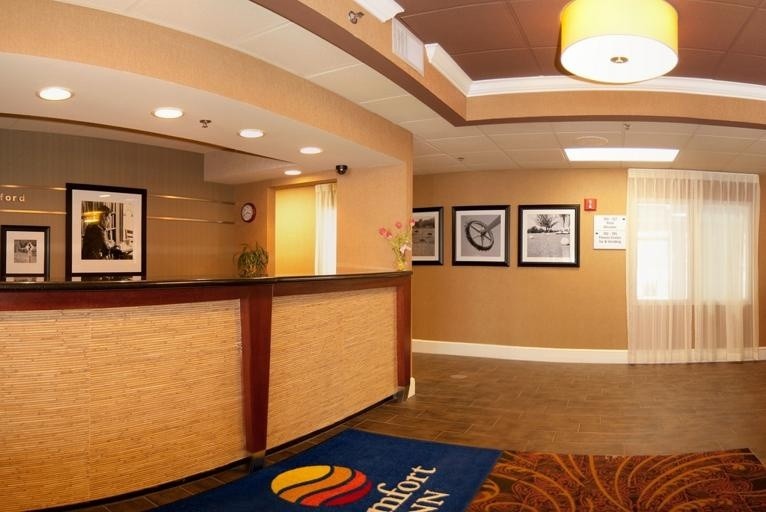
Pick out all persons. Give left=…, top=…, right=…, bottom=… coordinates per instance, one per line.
left=83, top=205, right=116, bottom=260
left=26, top=242, right=35, bottom=262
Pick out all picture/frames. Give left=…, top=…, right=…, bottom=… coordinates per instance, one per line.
left=0, top=223, right=50, bottom=282
left=64, top=182, right=146, bottom=283
left=411, top=206, right=444, bottom=266
left=517, top=203, right=581, bottom=268
left=451, top=203, right=512, bottom=266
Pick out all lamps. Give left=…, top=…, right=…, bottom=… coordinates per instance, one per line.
left=559, top=0, right=678, bottom=83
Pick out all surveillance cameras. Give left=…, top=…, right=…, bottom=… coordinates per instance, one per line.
left=336, top=165, right=347, bottom=174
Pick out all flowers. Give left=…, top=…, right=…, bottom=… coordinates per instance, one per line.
left=378, top=216, right=418, bottom=271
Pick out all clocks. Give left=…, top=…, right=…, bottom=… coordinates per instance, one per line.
left=239, top=202, right=256, bottom=222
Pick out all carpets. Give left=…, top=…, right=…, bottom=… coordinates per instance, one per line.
left=148, top=426, right=507, bottom=511
left=466, top=449, right=765, bottom=512
